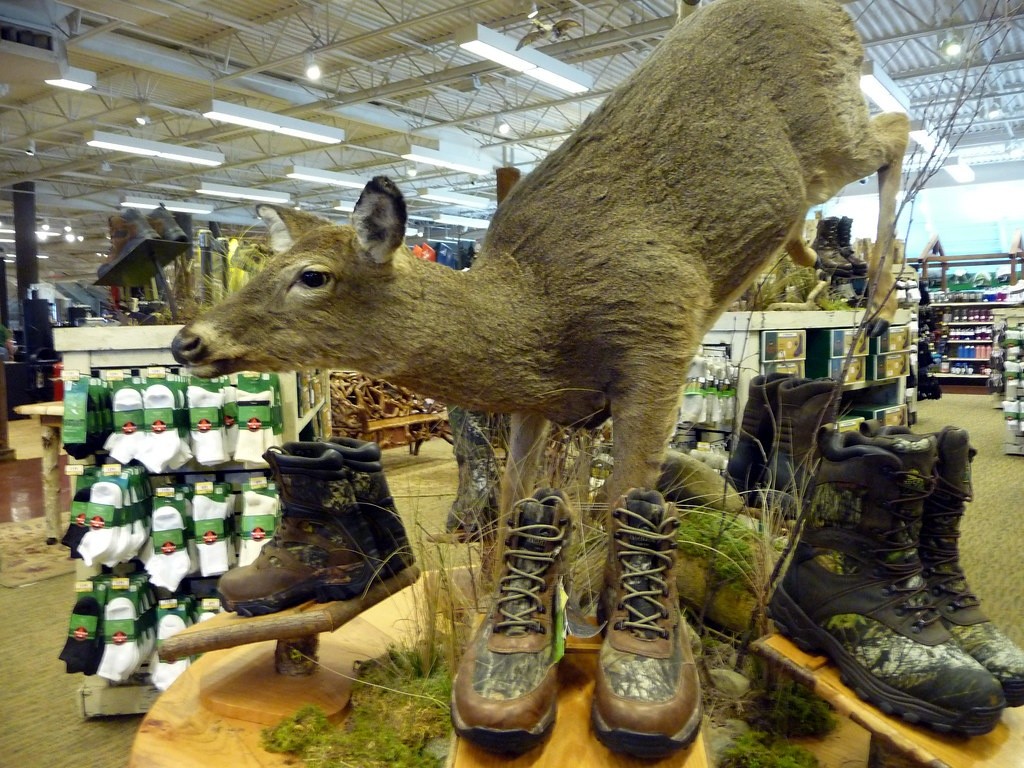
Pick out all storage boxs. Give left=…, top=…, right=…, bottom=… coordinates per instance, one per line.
left=759, top=326, right=911, bottom=433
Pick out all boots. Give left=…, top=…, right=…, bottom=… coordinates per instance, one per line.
left=451, top=489, right=581, bottom=753
left=446, top=401, right=503, bottom=529
left=753, top=379, right=841, bottom=516
left=592, top=485, right=702, bottom=755
left=839, top=216, right=867, bottom=272
left=812, top=217, right=854, bottom=277
left=859, top=418, right=1024, bottom=706
left=314, top=437, right=415, bottom=604
left=214, top=443, right=376, bottom=617
left=723, top=371, right=801, bottom=499
left=766, top=420, right=1004, bottom=736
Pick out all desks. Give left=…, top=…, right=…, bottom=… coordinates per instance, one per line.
left=11, top=402, right=67, bottom=537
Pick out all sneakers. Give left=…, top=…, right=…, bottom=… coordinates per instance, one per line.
left=146, top=202, right=187, bottom=241
left=96, top=208, right=162, bottom=278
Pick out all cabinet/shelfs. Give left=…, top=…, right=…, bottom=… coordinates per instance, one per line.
left=918, top=304, right=994, bottom=381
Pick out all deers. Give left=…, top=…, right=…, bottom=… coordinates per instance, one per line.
left=169, top=1, right=908, bottom=635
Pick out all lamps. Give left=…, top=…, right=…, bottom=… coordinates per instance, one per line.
left=39, top=23, right=593, bottom=231
